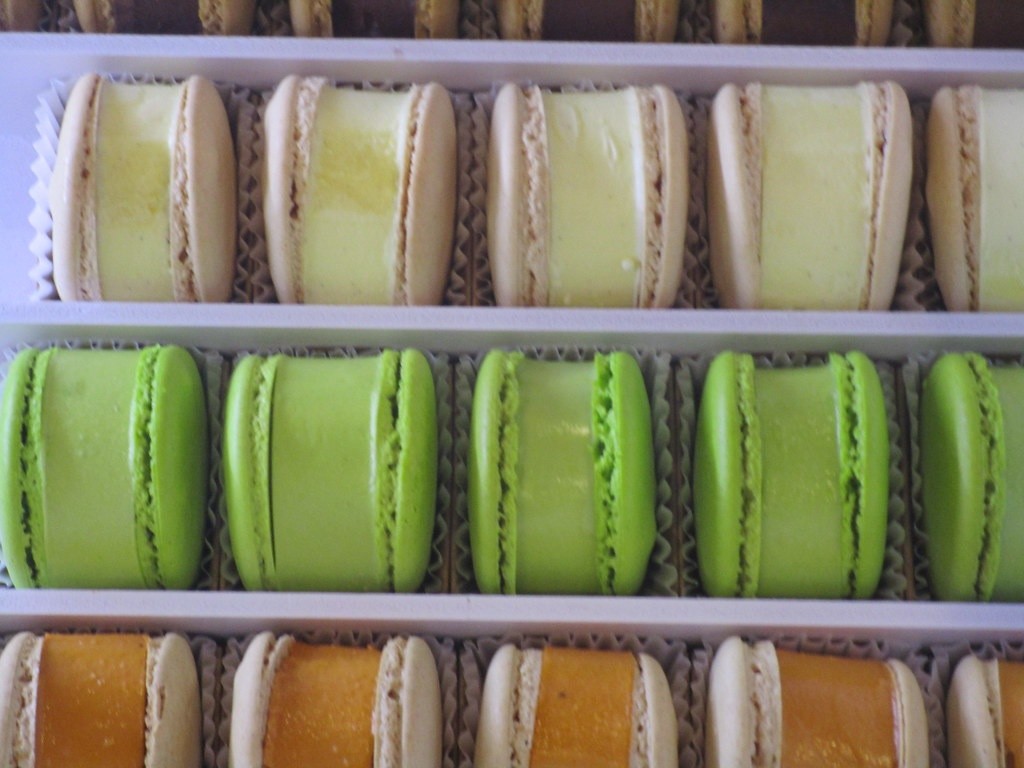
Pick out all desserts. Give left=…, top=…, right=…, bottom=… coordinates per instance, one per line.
left=0, top=0, right=1024, bottom=768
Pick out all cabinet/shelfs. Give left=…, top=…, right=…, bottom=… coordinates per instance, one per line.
left=0, top=0, right=1024, bottom=768
